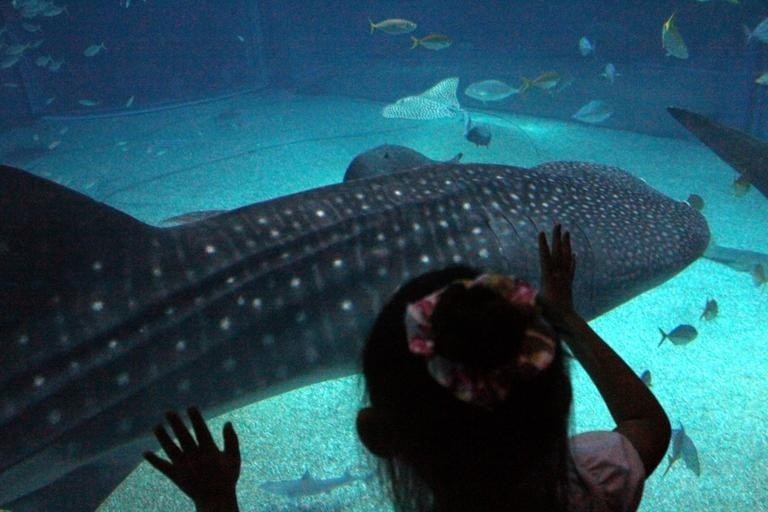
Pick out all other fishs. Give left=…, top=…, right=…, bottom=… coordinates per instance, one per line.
left=0, top=1, right=766, bottom=512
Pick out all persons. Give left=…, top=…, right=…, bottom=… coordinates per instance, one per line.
left=143, top=223, right=672, bottom=512
